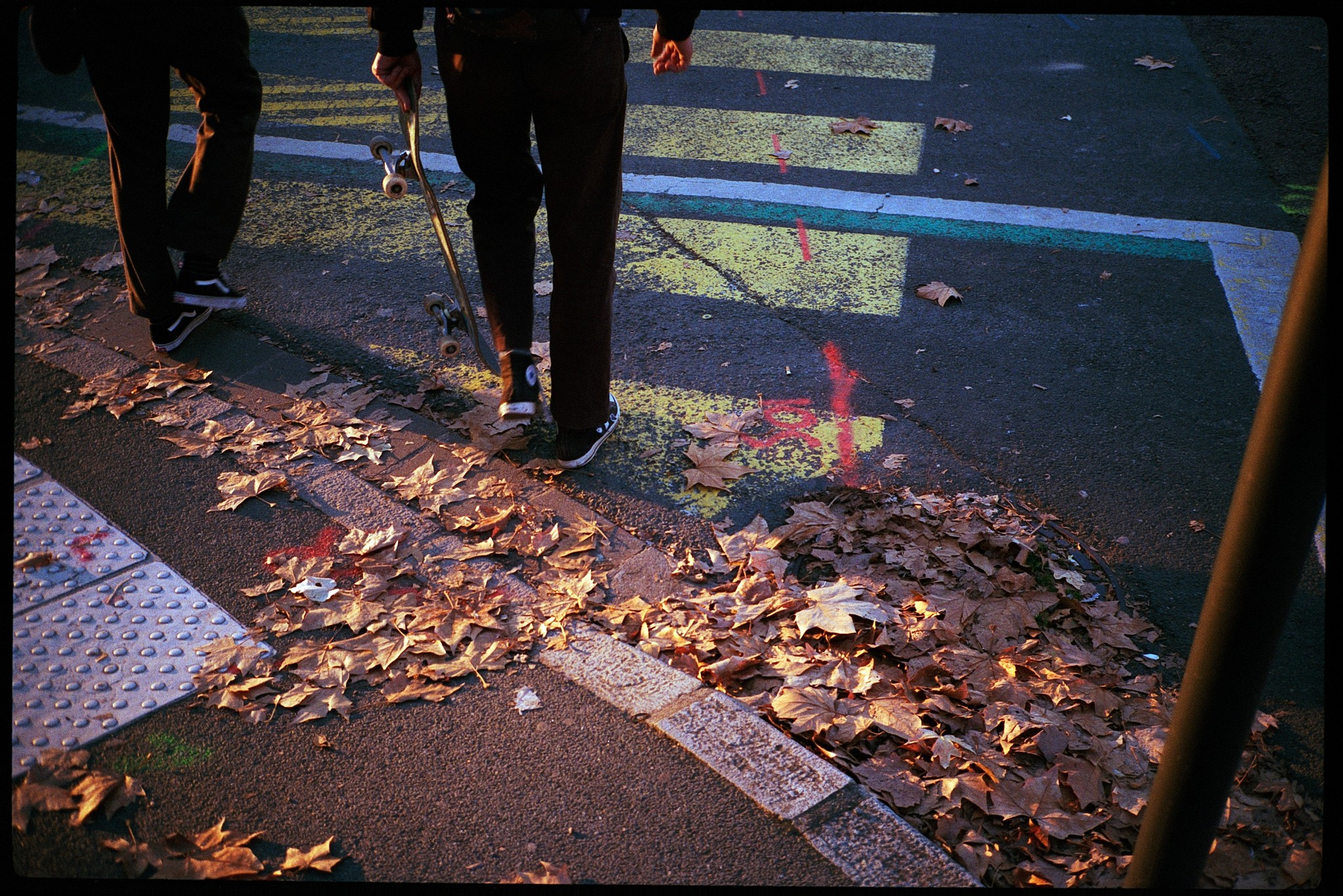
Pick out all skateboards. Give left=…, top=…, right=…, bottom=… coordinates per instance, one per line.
left=370, top=79, right=507, bottom=379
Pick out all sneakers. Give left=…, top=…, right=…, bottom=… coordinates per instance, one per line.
left=173, top=260, right=248, bottom=309
left=556, top=393, right=620, bottom=468
left=498, top=351, right=539, bottom=420
left=150, top=302, right=213, bottom=353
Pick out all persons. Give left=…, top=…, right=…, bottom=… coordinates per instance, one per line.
left=369, top=7, right=700, bottom=468
left=26, top=6, right=262, bottom=352
